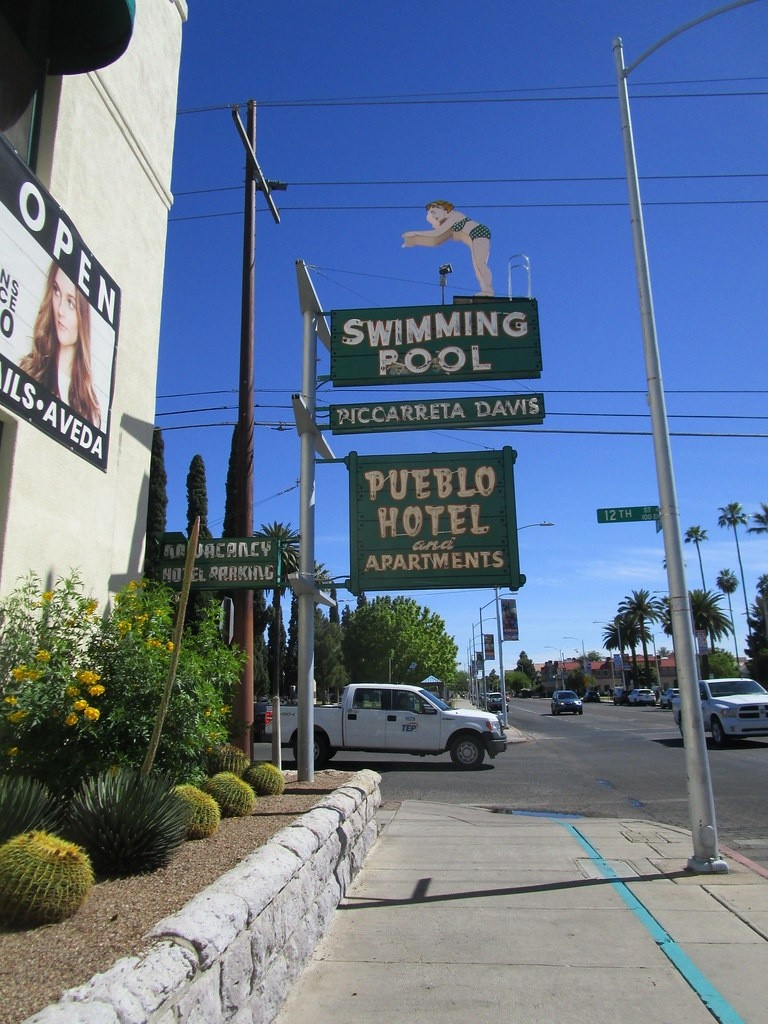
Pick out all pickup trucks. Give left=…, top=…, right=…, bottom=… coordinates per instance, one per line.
left=262, top=683, right=506, bottom=769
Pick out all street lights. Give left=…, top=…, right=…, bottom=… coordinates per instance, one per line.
left=513, top=663, right=523, bottom=672
left=653, top=590, right=702, bottom=681
left=543, top=645, right=565, bottom=691
left=495, top=520, right=554, bottom=726
left=594, top=621, right=626, bottom=689
left=468, top=591, right=519, bottom=707
left=563, top=636, right=589, bottom=692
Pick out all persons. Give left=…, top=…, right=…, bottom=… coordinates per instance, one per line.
left=16, top=259, right=103, bottom=431
left=396, top=693, right=419, bottom=713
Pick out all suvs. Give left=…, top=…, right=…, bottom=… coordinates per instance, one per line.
left=613, top=690, right=632, bottom=705
left=659, top=688, right=679, bottom=709
left=583, top=691, right=600, bottom=703
left=550, top=691, right=583, bottom=715
left=627, top=689, right=657, bottom=706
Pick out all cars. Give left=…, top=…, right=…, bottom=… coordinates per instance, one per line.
left=671, top=678, right=768, bottom=745
left=486, top=693, right=509, bottom=712
